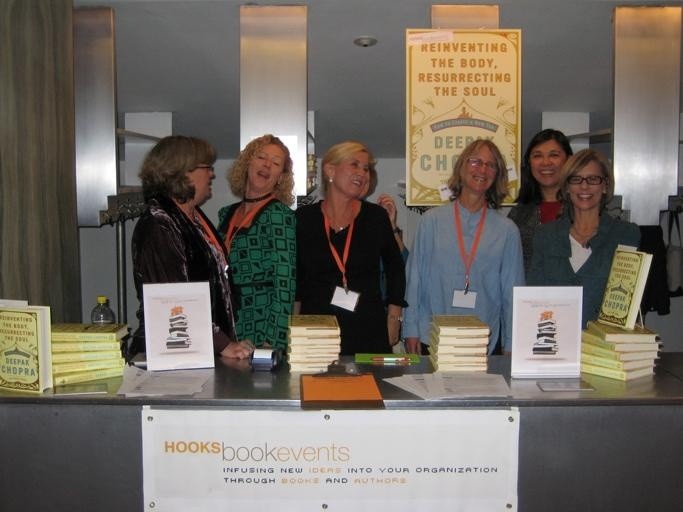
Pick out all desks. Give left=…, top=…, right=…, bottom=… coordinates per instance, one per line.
left=0, top=350, right=683, bottom=512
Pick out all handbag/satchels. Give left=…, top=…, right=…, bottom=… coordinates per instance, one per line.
left=666, top=210, right=683, bottom=298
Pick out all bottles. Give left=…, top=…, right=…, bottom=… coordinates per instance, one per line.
left=90, top=296, right=113, bottom=324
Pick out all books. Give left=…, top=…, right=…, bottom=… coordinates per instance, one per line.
left=597, top=248, right=653, bottom=331
left=427, top=315, right=491, bottom=372
left=51, top=323, right=130, bottom=387
left=287, top=314, right=342, bottom=374
left=1, top=306, right=53, bottom=394
left=581, top=318, right=665, bottom=382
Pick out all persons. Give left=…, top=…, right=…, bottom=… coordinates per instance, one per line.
left=129, top=135, right=255, bottom=360
left=377, top=193, right=404, bottom=255
left=403, top=139, right=526, bottom=357
left=295, top=142, right=406, bottom=356
left=526, top=151, right=641, bottom=329
left=506, top=128, right=573, bottom=269
left=217, top=134, right=296, bottom=359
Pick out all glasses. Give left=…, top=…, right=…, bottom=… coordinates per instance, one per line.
left=467, top=157, right=496, bottom=170
left=567, top=175, right=605, bottom=185
left=195, top=165, right=214, bottom=170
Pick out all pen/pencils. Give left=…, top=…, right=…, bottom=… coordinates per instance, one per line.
left=370, top=356, right=411, bottom=362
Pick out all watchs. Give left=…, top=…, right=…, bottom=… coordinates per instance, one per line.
left=385, top=314, right=404, bottom=324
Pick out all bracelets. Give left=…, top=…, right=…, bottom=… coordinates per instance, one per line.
left=392, top=227, right=401, bottom=235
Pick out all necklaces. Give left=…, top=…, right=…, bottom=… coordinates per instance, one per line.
left=243, top=192, right=273, bottom=204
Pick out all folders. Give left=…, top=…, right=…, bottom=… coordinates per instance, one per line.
left=301, top=360, right=386, bottom=410
